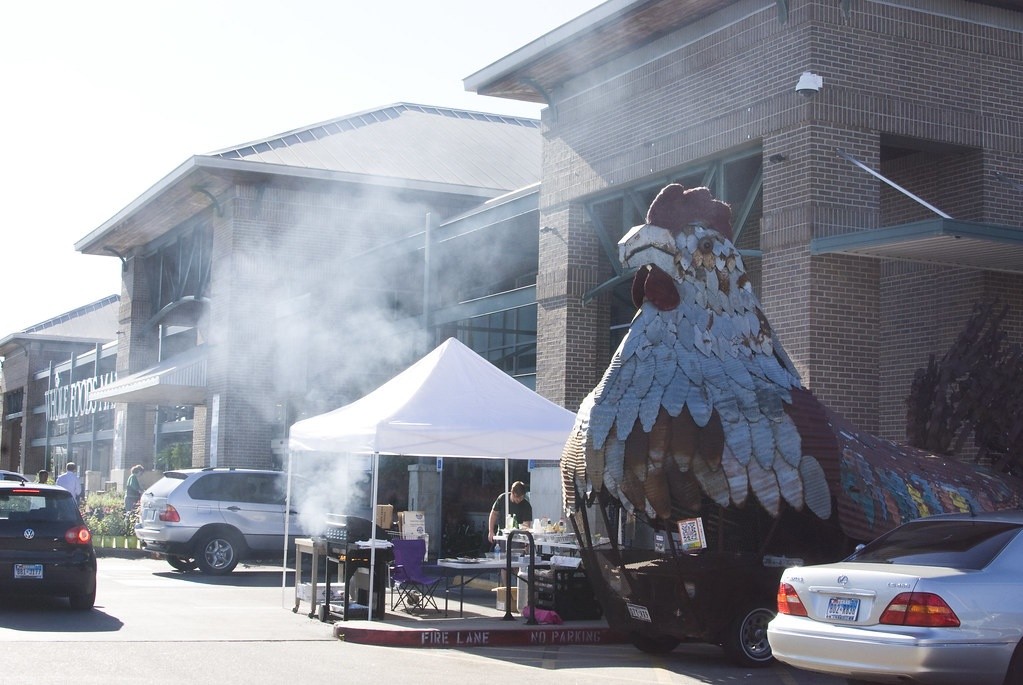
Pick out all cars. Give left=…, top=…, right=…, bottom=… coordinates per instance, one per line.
left=0, top=480, right=97, bottom=611
left=766, top=508, right=1022, bottom=685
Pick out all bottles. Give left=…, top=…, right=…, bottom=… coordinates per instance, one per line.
left=512, top=514, right=519, bottom=528
left=494, top=543, right=501, bottom=561
left=505, top=513, right=512, bottom=529
left=532, top=515, right=566, bottom=533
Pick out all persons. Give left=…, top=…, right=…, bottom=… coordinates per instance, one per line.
left=124, top=465, right=145, bottom=511
left=29, top=470, right=48, bottom=510
left=488, top=480, right=532, bottom=587
left=55, top=462, right=82, bottom=505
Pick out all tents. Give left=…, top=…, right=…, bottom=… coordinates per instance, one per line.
left=282, top=336, right=577, bottom=612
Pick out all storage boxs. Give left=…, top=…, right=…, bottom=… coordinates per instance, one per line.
left=375, top=504, right=394, bottom=529
left=491, top=587, right=520, bottom=613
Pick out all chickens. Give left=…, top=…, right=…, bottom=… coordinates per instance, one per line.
left=558, top=183, right=1023, bottom=667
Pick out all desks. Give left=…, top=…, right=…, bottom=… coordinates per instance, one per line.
left=437, top=559, right=547, bottom=618
left=490, top=533, right=610, bottom=559
left=292, top=538, right=343, bottom=619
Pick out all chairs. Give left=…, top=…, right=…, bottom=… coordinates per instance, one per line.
left=388, top=539, right=444, bottom=614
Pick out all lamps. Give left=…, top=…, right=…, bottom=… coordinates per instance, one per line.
left=540, top=225, right=555, bottom=233
left=769, top=154, right=787, bottom=164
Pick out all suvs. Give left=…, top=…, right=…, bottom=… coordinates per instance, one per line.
left=133, top=465, right=333, bottom=578
